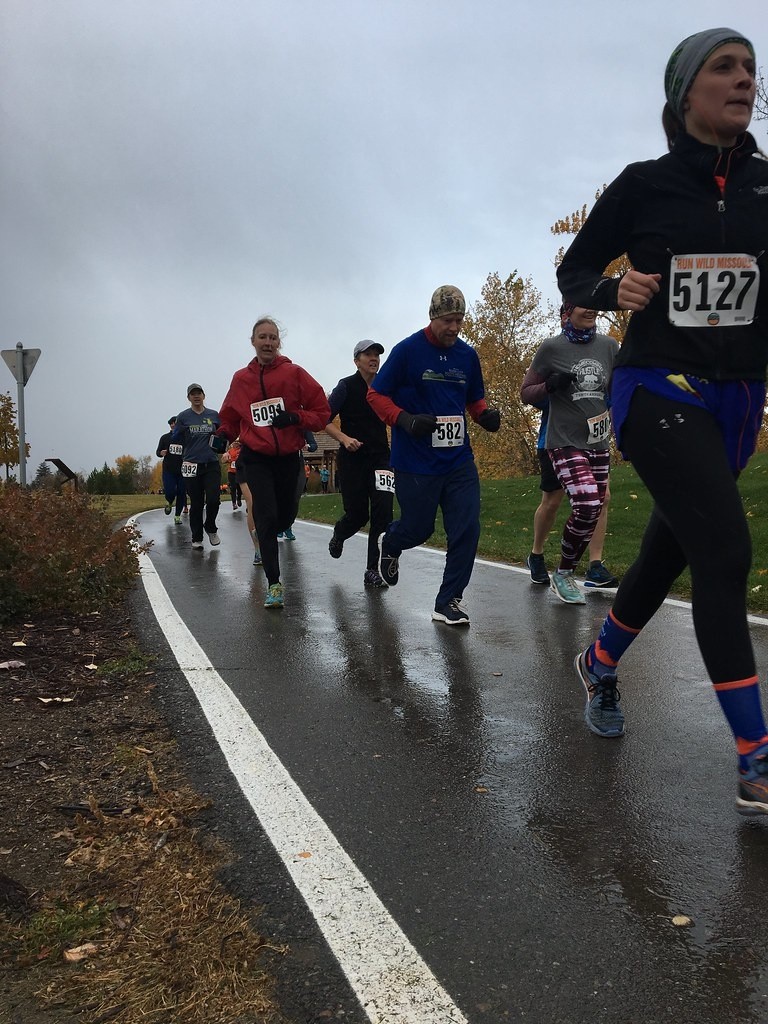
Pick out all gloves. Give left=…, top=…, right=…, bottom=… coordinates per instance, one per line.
left=212, top=432, right=226, bottom=455
left=545, top=371, right=576, bottom=391
left=479, top=409, right=499, bottom=431
left=397, top=411, right=438, bottom=439
left=271, top=408, right=299, bottom=429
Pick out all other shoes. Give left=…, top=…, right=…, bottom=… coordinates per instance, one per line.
left=238, top=499, right=241, bottom=507
left=285, top=528, right=297, bottom=540
left=233, top=503, right=237, bottom=510
left=277, top=530, right=283, bottom=538
left=253, top=553, right=262, bottom=564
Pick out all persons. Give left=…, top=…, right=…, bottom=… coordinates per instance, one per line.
left=519, top=292, right=621, bottom=605
left=556, top=26, right=768, bottom=816
left=168, top=384, right=222, bottom=550
left=366, top=285, right=501, bottom=625
left=134, top=479, right=231, bottom=496
left=324, top=341, right=394, bottom=587
left=207, top=318, right=341, bottom=608
left=156, top=416, right=187, bottom=525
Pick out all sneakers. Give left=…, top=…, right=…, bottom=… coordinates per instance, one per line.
left=165, top=501, right=221, bottom=551
left=574, top=645, right=625, bottom=738
left=264, top=582, right=284, bottom=607
left=527, top=552, right=550, bottom=585
left=734, top=756, right=767, bottom=817
left=551, top=570, right=587, bottom=604
left=252, top=529, right=258, bottom=542
left=377, top=532, right=402, bottom=587
left=431, top=599, right=472, bottom=624
left=364, top=567, right=389, bottom=588
left=584, top=565, right=619, bottom=588
left=328, top=522, right=345, bottom=559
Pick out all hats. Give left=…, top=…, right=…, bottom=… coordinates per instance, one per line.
left=562, top=293, right=577, bottom=317
left=187, top=384, right=204, bottom=395
left=353, top=340, right=385, bottom=359
left=429, top=285, right=466, bottom=323
left=665, top=28, right=755, bottom=124
